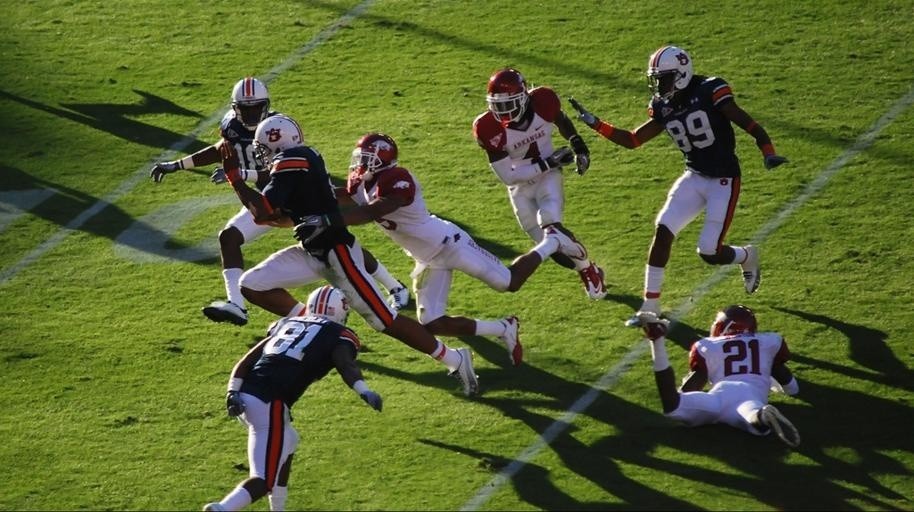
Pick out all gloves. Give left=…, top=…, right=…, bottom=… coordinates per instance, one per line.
left=569, top=136, right=592, bottom=176
left=224, top=388, right=246, bottom=418
left=356, top=389, right=384, bottom=413
left=291, top=212, right=336, bottom=244
left=149, top=161, right=182, bottom=185
left=545, top=144, right=575, bottom=174
left=210, top=167, right=229, bottom=186
left=763, top=152, right=790, bottom=173
left=569, top=96, right=598, bottom=127
left=219, top=140, right=241, bottom=177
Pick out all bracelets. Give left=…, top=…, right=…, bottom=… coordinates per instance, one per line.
left=761, top=141, right=776, bottom=158
left=240, top=167, right=258, bottom=185
left=599, top=118, right=614, bottom=139
left=227, top=375, right=244, bottom=394
left=353, top=379, right=369, bottom=395
left=177, top=151, right=196, bottom=171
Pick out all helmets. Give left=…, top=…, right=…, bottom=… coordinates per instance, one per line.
left=708, top=302, right=759, bottom=340
left=229, top=75, right=271, bottom=130
left=349, top=133, right=401, bottom=176
left=302, top=285, right=353, bottom=324
left=251, top=113, right=306, bottom=161
left=645, top=45, right=695, bottom=97
left=485, top=66, right=531, bottom=126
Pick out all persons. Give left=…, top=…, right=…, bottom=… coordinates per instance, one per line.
left=472, top=70, right=607, bottom=303
left=567, top=45, right=790, bottom=329
left=625, top=303, right=801, bottom=449
left=219, top=114, right=481, bottom=402
left=330, top=132, right=588, bottom=367
left=150, top=78, right=410, bottom=326
left=202, top=283, right=383, bottom=511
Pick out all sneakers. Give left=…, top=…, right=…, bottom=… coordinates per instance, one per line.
left=202, top=500, right=225, bottom=512
left=542, top=226, right=589, bottom=259
left=635, top=310, right=672, bottom=338
left=577, top=260, right=609, bottom=302
left=738, top=242, right=762, bottom=296
left=761, top=402, right=803, bottom=450
left=625, top=301, right=663, bottom=323
left=385, top=279, right=412, bottom=312
left=198, top=302, right=250, bottom=325
left=496, top=313, right=524, bottom=370
left=447, top=345, right=481, bottom=398
